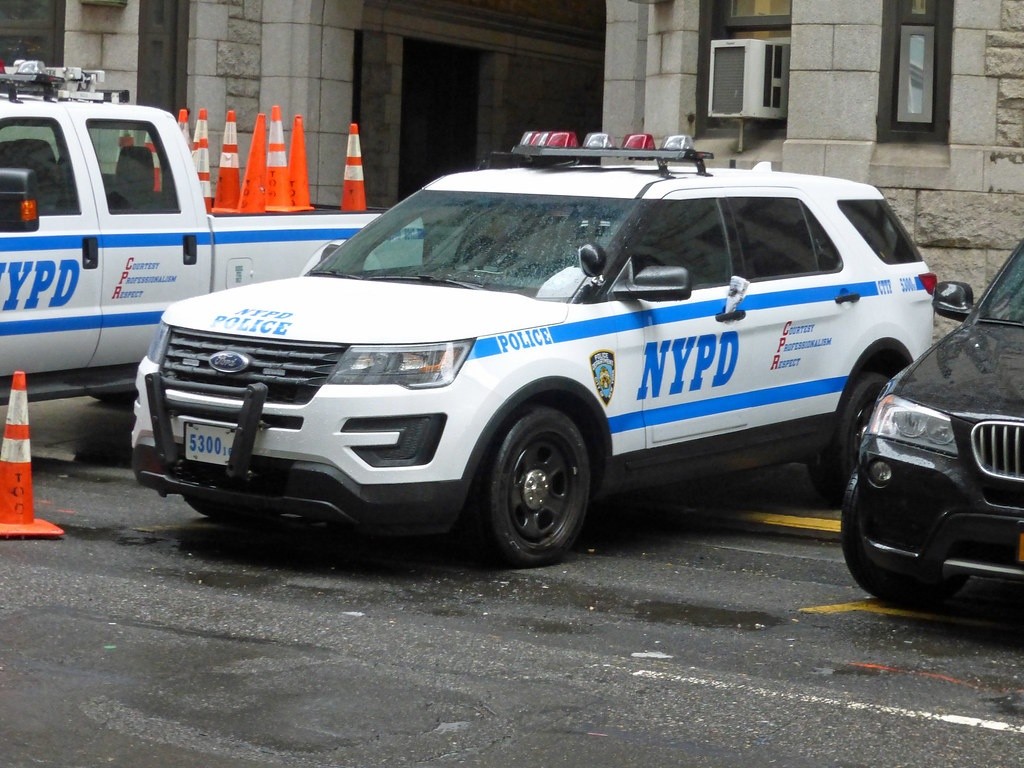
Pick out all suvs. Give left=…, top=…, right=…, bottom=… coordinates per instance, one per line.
left=131, top=130, right=938, bottom=567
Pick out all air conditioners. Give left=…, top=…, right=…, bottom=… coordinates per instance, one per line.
left=708, top=37, right=792, bottom=153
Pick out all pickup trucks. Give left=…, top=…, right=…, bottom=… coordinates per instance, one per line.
left=2, top=58, right=426, bottom=410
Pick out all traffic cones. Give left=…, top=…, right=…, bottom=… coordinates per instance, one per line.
left=267, top=106, right=299, bottom=212
left=1, top=371, right=67, bottom=539
left=237, top=113, right=267, bottom=214
left=193, top=108, right=213, bottom=214
left=342, top=124, right=367, bottom=213
left=289, top=115, right=316, bottom=212
left=117, top=130, right=136, bottom=162
left=211, top=110, right=240, bottom=215
left=145, top=128, right=160, bottom=193
left=178, top=109, right=192, bottom=150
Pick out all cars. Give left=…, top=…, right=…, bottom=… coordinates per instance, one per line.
left=842, top=239, right=1024, bottom=621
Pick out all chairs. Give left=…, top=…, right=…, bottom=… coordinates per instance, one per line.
left=114, top=146, right=155, bottom=193
left=0, top=138, right=63, bottom=204
